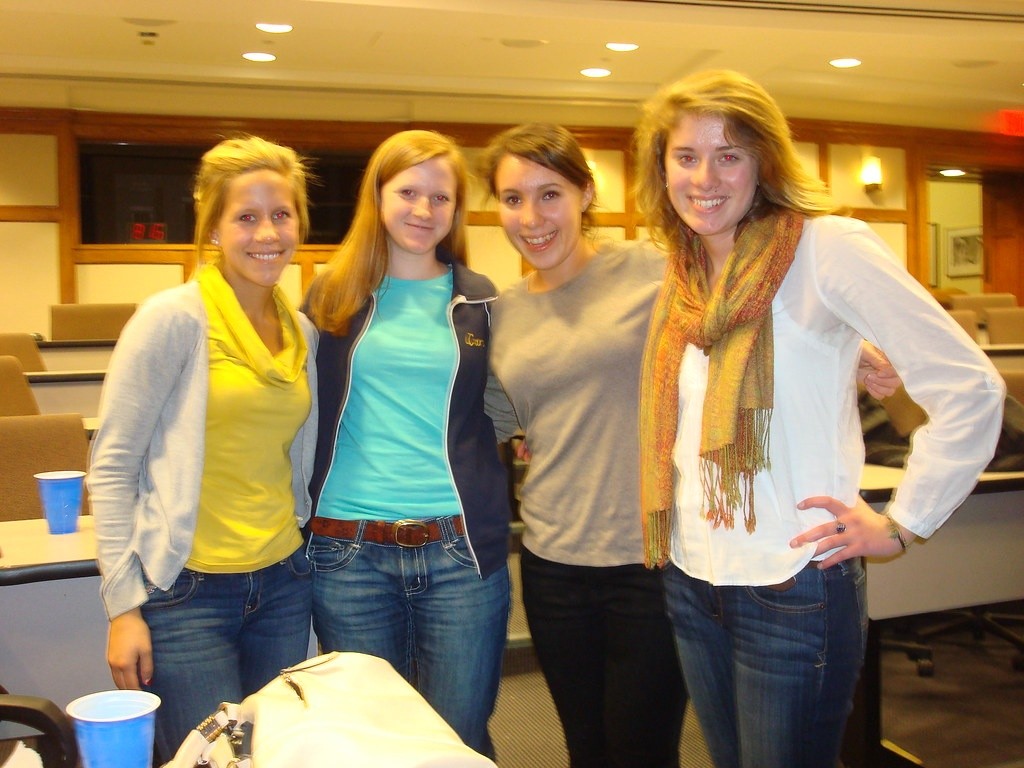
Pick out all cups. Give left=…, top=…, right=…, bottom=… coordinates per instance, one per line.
left=66, top=690, right=161, bottom=768
left=34, top=470, right=87, bottom=534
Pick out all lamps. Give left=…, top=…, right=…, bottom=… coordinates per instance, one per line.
left=862, top=157, right=882, bottom=192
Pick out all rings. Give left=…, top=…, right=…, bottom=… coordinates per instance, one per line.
left=836, top=519, right=846, bottom=534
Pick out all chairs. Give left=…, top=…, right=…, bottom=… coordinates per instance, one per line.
left=0, top=301, right=139, bottom=523
left=929, top=286, right=1024, bottom=344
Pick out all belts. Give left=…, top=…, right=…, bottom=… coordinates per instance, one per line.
left=306, top=515, right=463, bottom=548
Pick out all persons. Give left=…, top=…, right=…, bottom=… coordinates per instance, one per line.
left=480, top=121, right=903, bottom=768
left=632, top=67, right=1005, bottom=768
left=300, top=130, right=511, bottom=762
left=86, top=136, right=320, bottom=768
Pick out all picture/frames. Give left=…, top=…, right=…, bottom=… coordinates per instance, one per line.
left=945, top=224, right=983, bottom=278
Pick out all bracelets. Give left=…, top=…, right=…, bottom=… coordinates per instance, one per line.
left=884, top=514, right=909, bottom=550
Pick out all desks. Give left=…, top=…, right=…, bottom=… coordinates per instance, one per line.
left=0, top=518, right=317, bottom=741
left=860, top=461, right=1024, bottom=768
left=24, top=370, right=106, bottom=418
left=980, top=345, right=1024, bottom=373
left=82, top=417, right=98, bottom=440
left=35, top=338, right=118, bottom=371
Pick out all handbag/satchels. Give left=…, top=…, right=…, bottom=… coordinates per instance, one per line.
left=162, top=652, right=499, bottom=768
